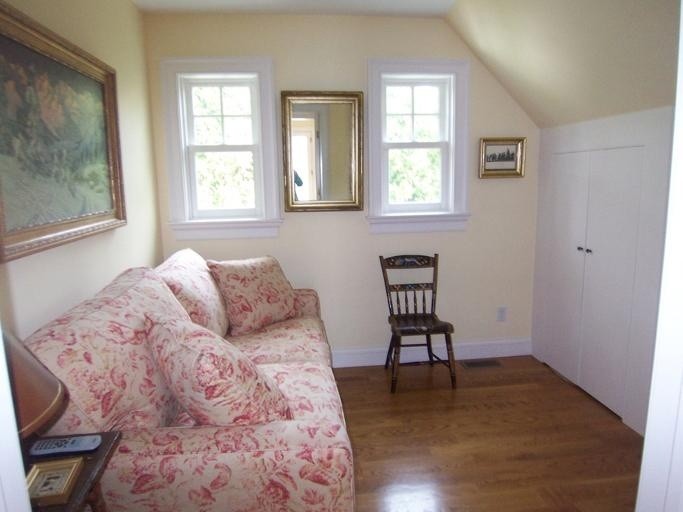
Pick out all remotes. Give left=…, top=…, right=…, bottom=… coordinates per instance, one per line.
left=29, top=434, right=102, bottom=457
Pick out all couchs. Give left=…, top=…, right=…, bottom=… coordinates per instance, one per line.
left=19, top=248, right=356, bottom=512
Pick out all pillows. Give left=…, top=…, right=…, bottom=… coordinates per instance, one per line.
left=206, top=256, right=303, bottom=335
left=142, top=310, right=291, bottom=424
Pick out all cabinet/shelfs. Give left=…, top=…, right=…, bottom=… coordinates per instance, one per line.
left=541, top=143, right=647, bottom=423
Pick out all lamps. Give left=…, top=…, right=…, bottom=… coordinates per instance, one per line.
left=0, top=322, right=71, bottom=444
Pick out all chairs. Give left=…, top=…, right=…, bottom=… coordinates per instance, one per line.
left=379, top=252, right=456, bottom=393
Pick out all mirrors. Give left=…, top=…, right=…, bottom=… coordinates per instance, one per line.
left=281, top=91, right=364, bottom=213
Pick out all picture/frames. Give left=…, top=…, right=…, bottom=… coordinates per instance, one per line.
left=0, top=1, right=128, bottom=263
left=25, top=456, right=83, bottom=506
left=480, top=137, right=527, bottom=178
